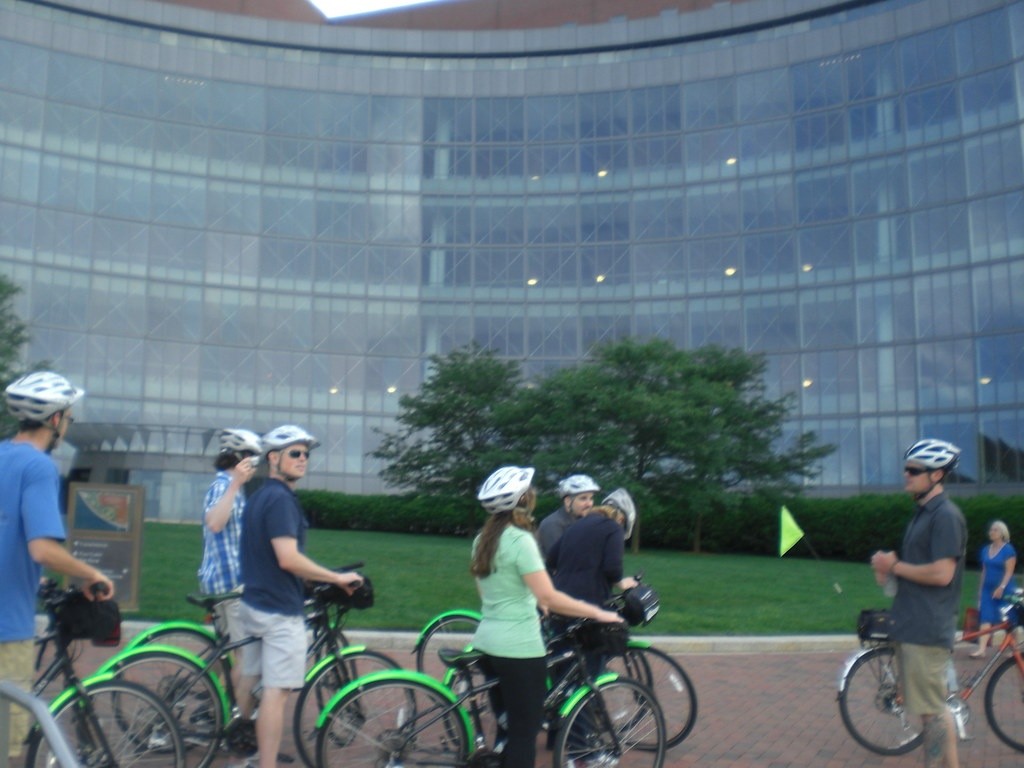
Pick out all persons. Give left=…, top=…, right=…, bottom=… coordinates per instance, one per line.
left=871, top=439, right=970, bottom=768
left=471, top=464, right=625, bottom=768
left=229, top=424, right=363, bottom=768
left=0, top=372, right=114, bottom=768
left=544, top=487, right=638, bottom=768
left=534, top=474, right=600, bottom=568
left=198, top=429, right=262, bottom=714
left=969, top=520, right=1022, bottom=658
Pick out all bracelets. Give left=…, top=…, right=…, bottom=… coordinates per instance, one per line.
left=1000, top=585, right=1006, bottom=589
left=890, top=560, right=903, bottom=577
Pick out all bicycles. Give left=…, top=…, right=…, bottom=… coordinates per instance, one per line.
left=0, top=562, right=698, bottom=768
left=834, top=587, right=1024, bottom=757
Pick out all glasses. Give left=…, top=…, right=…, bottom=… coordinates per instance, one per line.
left=903, top=465, right=930, bottom=476
left=64, top=415, right=76, bottom=424
left=287, top=450, right=312, bottom=459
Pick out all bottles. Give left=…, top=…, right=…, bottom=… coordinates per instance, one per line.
left=947, top=657, right=958, bottom=692
left=883, top=574, right=897, bottom=598
left=252, top=679, right=262, bottom=700
left=498, top=711, right=508, bottom=731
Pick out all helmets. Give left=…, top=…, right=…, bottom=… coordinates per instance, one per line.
left=2, top=370, right=85, bottom=422
left=600, top=489, right=637, bottom=541
left=558, top=474, right=601, bottom=499
left=902, top=438, right=963, bottom=475
left=218, top=429, right=264, bottom=454
left=475, top=464, right=536, bottom=516
left=259, top=425, right=321, bottom=455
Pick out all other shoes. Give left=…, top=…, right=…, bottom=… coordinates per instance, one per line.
left=968, top=650, right=989, bottom=660
left=491, top=733, right=509, bottom=753
left=566, top=752, right=620, bottom=768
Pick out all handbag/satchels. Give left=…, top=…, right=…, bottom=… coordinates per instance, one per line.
left=963, top=608, right=998, bottom=645
left=854, top=606, right=897, bottom=643
